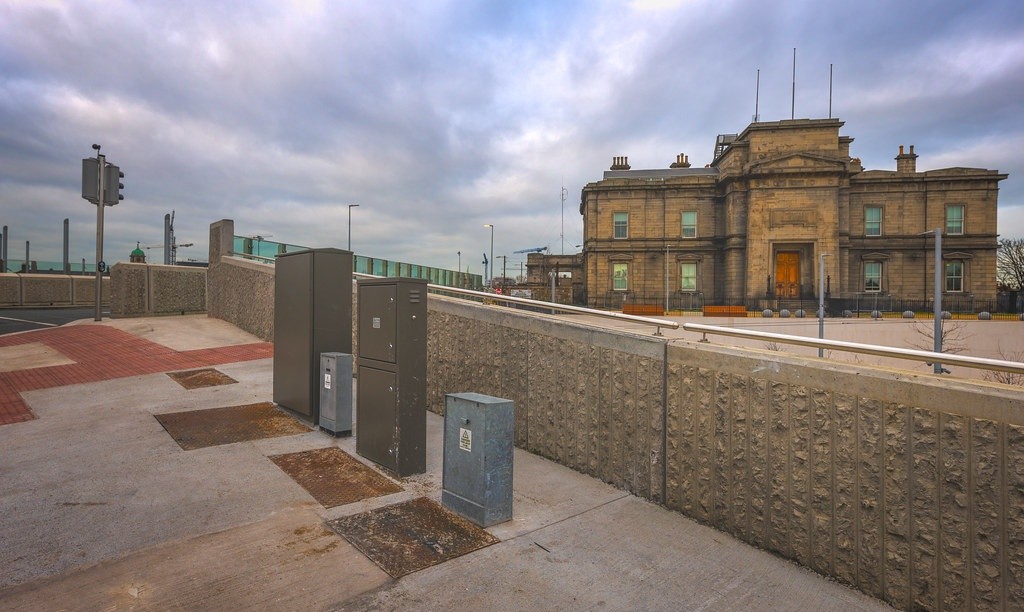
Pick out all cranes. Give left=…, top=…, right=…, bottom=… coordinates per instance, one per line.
left=140, top=210, right=194, bottom=266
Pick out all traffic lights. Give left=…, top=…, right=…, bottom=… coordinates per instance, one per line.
left=103, top=165, right=125, bottom=207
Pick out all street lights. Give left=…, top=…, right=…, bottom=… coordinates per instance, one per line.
left=819, top=253, right=831, bottom=358
left=348, top=204, right=360, bottom=251
left=666, top=245, right=672, bottom=315
left=549, top=269, right=556, bottom=315
left=457, top=251, right=461, bottom=288
left=918, top=227, right=943, bottom=374
left=484, top=224, right=493, bottom=288
